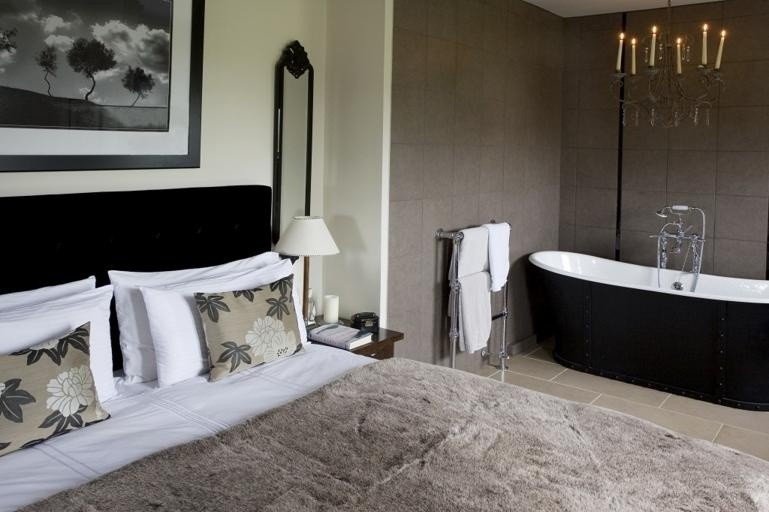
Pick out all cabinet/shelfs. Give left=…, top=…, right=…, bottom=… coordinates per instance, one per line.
left=433, top=213, right=513, bottom=377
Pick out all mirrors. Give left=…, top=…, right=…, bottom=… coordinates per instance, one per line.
left=271, top=36, right=317, bottom=263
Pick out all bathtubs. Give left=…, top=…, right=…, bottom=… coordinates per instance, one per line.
left=528, top=250, right=769, bottom=412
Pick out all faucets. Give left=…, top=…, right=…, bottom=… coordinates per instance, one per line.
left=670, top=233, right=682, bottom=256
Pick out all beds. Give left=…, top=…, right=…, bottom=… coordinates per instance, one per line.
left=0, top=184, right=767, bottom=510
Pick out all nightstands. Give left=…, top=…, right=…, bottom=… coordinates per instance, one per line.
left=304, top=314, right=405, bottom=361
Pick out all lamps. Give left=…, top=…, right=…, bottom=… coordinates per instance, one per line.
left=602, top=0, right=732, bottom=130
left=272, top=216, right=343, bottom=328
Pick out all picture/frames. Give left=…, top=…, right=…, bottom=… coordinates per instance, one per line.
left=2, top=0, right=205, bottom=172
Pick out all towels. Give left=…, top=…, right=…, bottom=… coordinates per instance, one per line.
left=455, top=272, right=493, bottom=353
left=488, top=221, right=509, bottom=292
left=450, top=225, right=490, bottom=318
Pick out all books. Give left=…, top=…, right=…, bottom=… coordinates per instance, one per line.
left=308, top=323, right=374, bottom=351
